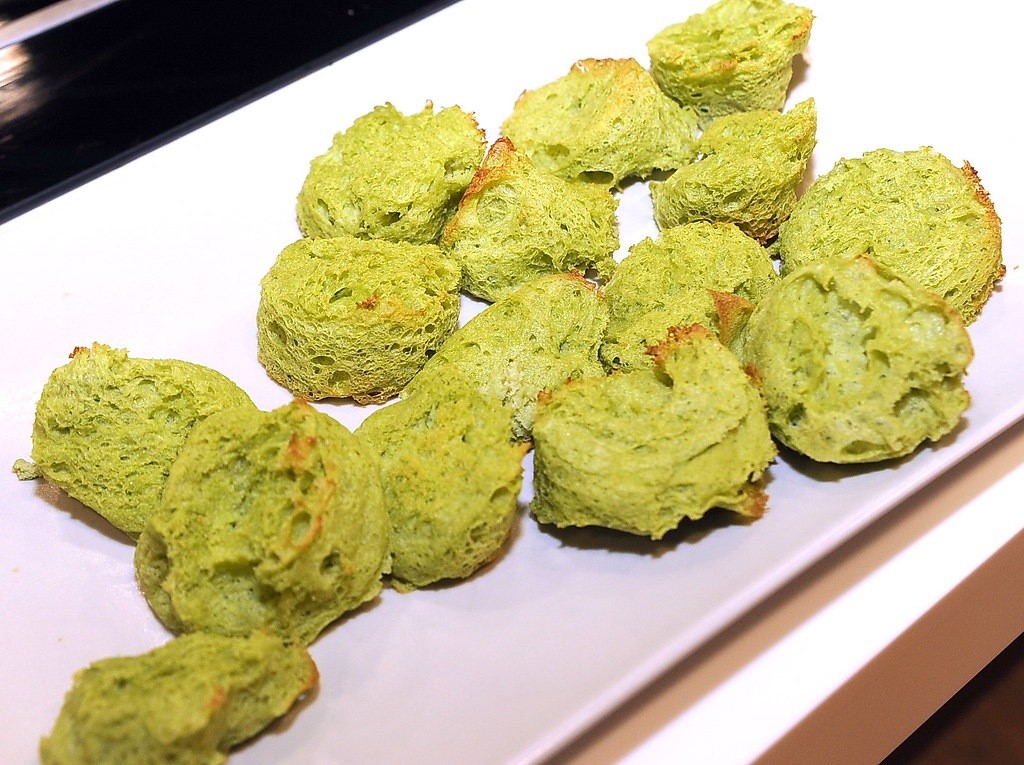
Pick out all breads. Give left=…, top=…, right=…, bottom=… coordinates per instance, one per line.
left=17, top=1, right=1002, bottom=764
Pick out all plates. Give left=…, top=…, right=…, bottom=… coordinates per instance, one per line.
left=0, top=1, right=1023, bottom=764
